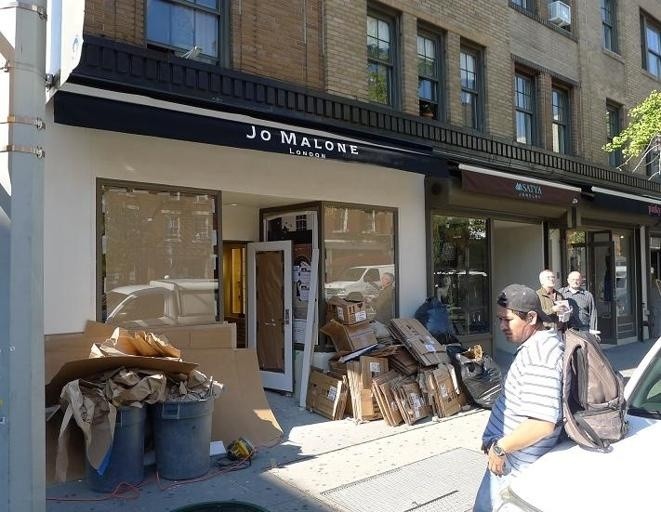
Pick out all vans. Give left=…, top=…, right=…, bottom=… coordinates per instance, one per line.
left=323, top=264, right=393, bottom=302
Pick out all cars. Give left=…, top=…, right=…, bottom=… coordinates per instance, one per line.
left=492, top=327, right=661, bottom=512
left=616, top=266, right=628, bottom=312
left=433, top=270, right=487, bottom=299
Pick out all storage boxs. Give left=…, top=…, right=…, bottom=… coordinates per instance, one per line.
left=319, top=291, right=378, bottom=358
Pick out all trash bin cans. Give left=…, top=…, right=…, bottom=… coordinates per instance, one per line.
left=83, top=393, right=215, bottom=492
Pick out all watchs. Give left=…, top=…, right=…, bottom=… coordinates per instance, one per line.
left=491, top=440, right=506, bottom=458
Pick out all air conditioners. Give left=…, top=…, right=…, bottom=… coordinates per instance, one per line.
left=547, top=2, right=573, bottom=28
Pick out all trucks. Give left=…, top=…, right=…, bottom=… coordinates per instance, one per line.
left=103, top=278, right=217, bottom=325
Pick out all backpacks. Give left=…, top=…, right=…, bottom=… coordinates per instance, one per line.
left=562, top=328, right=628, bottom=453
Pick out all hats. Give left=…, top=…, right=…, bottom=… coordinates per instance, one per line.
left=496, top=283, right=553, bottom=322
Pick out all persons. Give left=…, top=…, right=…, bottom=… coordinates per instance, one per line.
left=536, top=270, right=597, bottom=331
left=473, top=282, right=571, bottom=512
left=436, top=274, right=456, bottom=304
left=369, top=273, right=396, bottom=323
